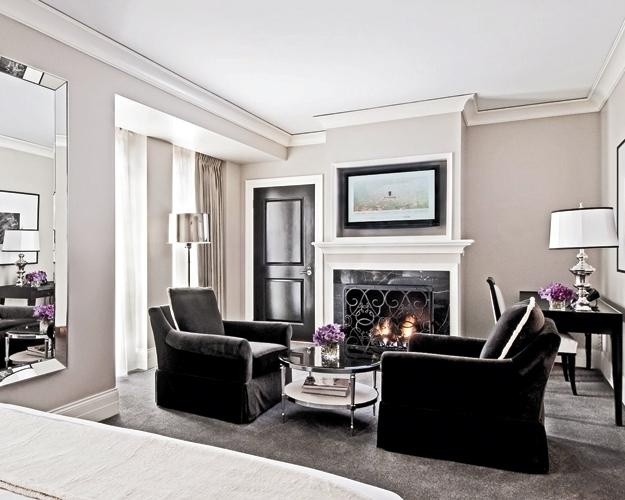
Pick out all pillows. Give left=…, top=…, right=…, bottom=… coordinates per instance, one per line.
left=165, top=287, right=230, bottom=337
left=478, top=292, right=547, bottom=361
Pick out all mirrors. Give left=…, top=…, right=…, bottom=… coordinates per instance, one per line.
left=0, top=58, right=71, bottom=392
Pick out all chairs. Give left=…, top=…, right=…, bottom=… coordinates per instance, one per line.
left=148, top=302, right=296, bottom=428
left=0, top=303, right=48, bottom=369
left=372, top=313, right=558, bottom=477
left=485, top=275, right=582, bottom=398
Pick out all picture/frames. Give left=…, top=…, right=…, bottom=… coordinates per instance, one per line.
left=614, top=138, right=625, bottom=273
left=0, top=189, right=41, bottom=267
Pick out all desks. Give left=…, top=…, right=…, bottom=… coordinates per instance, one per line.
left=2, top=321, right=56, bottom=372
left=0, top=280, right=54, bottom=308
left=516, top=288, right=625, bottom=427
left=277, top=344, right=383, bottom=438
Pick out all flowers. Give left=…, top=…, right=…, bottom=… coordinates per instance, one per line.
left=31, top=303, right=55, bottom=320
left=24, top=269, right=47, bottom=284
left=536, top=281, right=579, bottom=304
left=312, top=322, right=346, bottom=346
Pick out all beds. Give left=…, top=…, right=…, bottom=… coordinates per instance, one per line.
left=0, top=400, right=407, bottom=500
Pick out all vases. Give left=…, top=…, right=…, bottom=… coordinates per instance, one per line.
left=549, top=299, right=566, bottom=310
left=320, top=342, right=338, bottom=361
left=38, top=319, right=50, bottom=332
left=30, top=281, right=42, bottom=288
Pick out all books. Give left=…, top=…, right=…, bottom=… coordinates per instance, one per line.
left=301, top=377, right=350, bottom=396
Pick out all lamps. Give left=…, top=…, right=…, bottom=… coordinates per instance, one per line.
left=0, top=228, right=42, bottom=288
left=163, top=211, right=214, bottom=288
left=546, top=200, right=621, bottom=312
